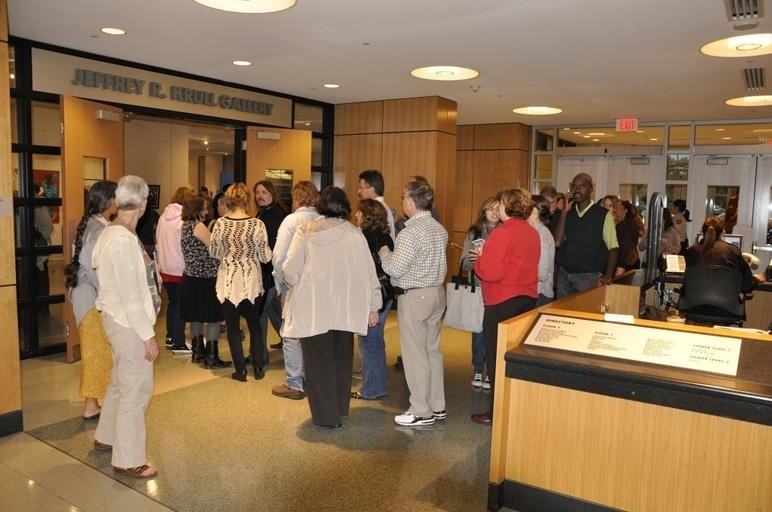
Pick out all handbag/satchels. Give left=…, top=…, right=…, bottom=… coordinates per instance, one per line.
left=442, top=258, right=485, bottom=334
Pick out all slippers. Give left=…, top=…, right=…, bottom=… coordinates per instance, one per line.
left=84, top=413, right=99, bottom=420
left=93, top=440, right=112, bottom=450
left=113, top=465, right=156, bottom=478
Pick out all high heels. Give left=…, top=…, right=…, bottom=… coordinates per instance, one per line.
left=350, top=391, right=363, bottom=399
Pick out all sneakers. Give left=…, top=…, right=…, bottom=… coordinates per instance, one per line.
left=483, top=376, right=491, bottom=389
left=472, top=412, right=492, bottom=424
left=394, top=411, right=435, bottom=426
left=433, top=410, right=447, bottom=419
left=172, top=343, right=192, bottom=353
left=165, top=334, right=173, bottom=347
left=471, top=372, right=483, bottom=387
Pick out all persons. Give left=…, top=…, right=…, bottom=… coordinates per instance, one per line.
left=394, top=176, right=448, bottom=371
left=552, top=174, right=688, bottom=299
left=91, top=175, right=162, bottom=479
left=460, top=188, right=555, bottom=423
left=677, top=215, right=754, bottom=324
left=358, top=170, right=395, bottom=242
left=64, top=180, right=122, bottom=421
left=136, top=181, right=288, bottom=381
left=281, top=186, right=383, bottom=430
left=351, top=199, right=394, bottom=399
left=271, top=181, right=326, bottom=400
left=378, top=181, right=447, bottom=427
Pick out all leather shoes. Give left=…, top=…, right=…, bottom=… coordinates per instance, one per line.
left=270, top=342, right=283, bottom=349
left=232, top=371, right=246, bottom=381
left=245, top=354, right=269, bottom=365
left=272, top=385, right=303, bottom=399
left=254, top=368, right=264, bottom=380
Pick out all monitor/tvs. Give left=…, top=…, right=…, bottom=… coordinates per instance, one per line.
left=697, top=234, right=742, bottom=250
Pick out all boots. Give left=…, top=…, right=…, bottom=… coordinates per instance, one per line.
left=192, top=334, right=206, bottom=362
left=205, top=340, right=233, bottom=368
